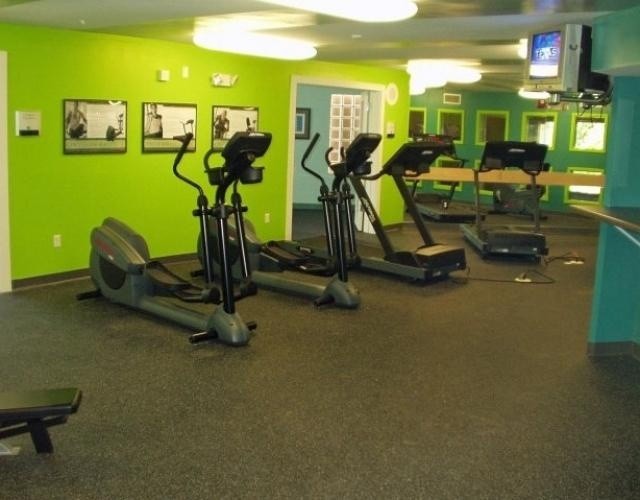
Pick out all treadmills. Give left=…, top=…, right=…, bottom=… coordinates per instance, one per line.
left=412, top=151, right=489, bottom=220
left=296, top=136, right=466, bottom=282
left=460, top=140, right=549, bottom=262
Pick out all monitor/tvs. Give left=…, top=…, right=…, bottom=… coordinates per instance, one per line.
left=524, top=24, right=592, bottom=93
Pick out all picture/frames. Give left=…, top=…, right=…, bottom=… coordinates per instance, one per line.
left=62, top=96, right=260, bottom=156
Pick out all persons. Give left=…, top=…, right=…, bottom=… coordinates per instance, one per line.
left=146, top=104, right=163, bottom=139
left=213, top=109, right=229, bottom=139
left=66, top=101, right=88, bottom=139
